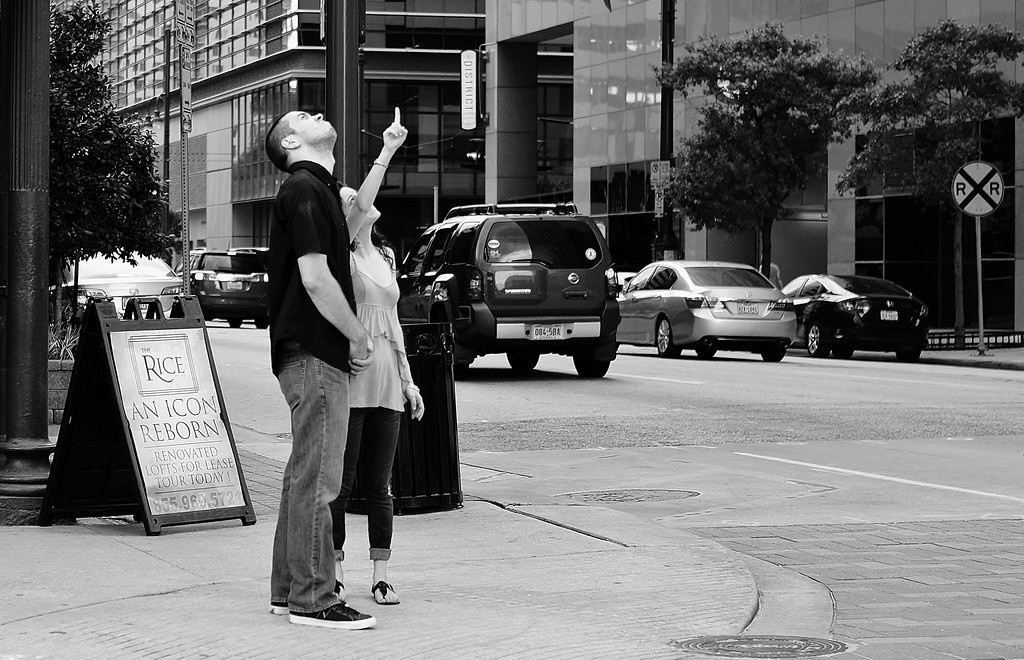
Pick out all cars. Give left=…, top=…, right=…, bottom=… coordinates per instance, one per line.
left=612, top=259, right=799, bottom=362
left=48, top=247, right=184, bottom=320
left=781, top=274, right=931, bottom=362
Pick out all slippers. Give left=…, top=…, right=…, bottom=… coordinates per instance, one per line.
left=333, top=579, right=347, bottom=601
left=372, top=581, right=400, bottom=605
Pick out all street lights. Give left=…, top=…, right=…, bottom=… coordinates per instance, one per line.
left=146, top=21, right=173, bottom=233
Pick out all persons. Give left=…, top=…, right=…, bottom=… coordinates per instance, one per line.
left=336, top=106, right=425, bottom=607
left=266, top=107, right=378, bottom=630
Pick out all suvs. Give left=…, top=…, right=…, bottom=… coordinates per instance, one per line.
left=175, top=248, right=273, bottom=329
left=397, top=203, right=623, bottom=378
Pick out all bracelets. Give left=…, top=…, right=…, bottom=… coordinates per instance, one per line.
left=407, top=382, right=420, bottom=392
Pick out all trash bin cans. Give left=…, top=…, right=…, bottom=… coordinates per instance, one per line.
left=345, top=321, right=464, bottom=515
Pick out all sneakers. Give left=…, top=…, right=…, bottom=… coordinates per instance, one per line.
left=289, top=600, right=377, bottom=630
left=270, top=598, right=348, bottom=615
